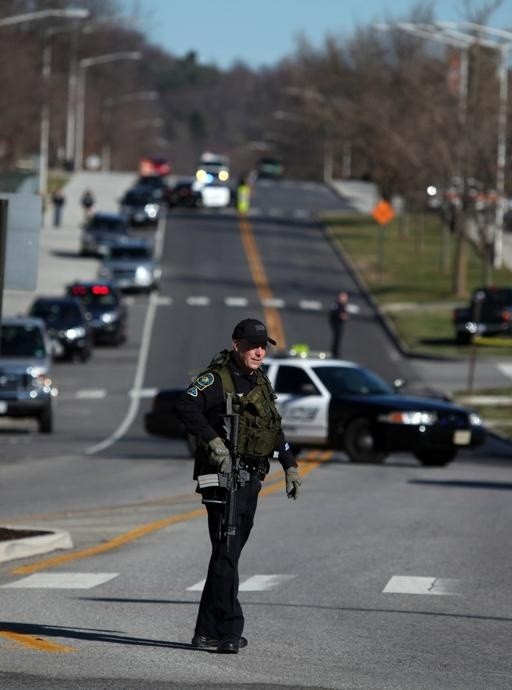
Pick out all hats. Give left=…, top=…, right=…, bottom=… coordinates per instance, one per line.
left=232, top=318, right=278, bottom=347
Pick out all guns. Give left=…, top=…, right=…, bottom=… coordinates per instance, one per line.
left=197, top=414, right=241, bottom=553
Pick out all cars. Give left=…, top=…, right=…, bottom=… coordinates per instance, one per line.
left=143, top=345, right=487, bottom=466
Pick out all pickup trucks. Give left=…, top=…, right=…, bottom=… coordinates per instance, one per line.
left=454, top=285, right=511, bottom=344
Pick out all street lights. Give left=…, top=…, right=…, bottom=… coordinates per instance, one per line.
left=1, top=7, right=169, bottom=197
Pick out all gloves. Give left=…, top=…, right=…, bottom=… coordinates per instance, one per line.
left=284, top=467, right=302, bottom=501
left=206, top=436, right=233, bottom=476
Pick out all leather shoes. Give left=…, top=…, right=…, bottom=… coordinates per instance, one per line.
left=190, top=631, right=249, bottom=654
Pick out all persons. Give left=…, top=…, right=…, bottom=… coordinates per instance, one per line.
left=81, top=190, right=93, bottom=218
left=172, top=318, right=303, bottom=653
left=329, top=291, right=349, bottom=358
left=52, top=191, right=65, bottom=227
left=235, top=178, right=250, bottom=221
